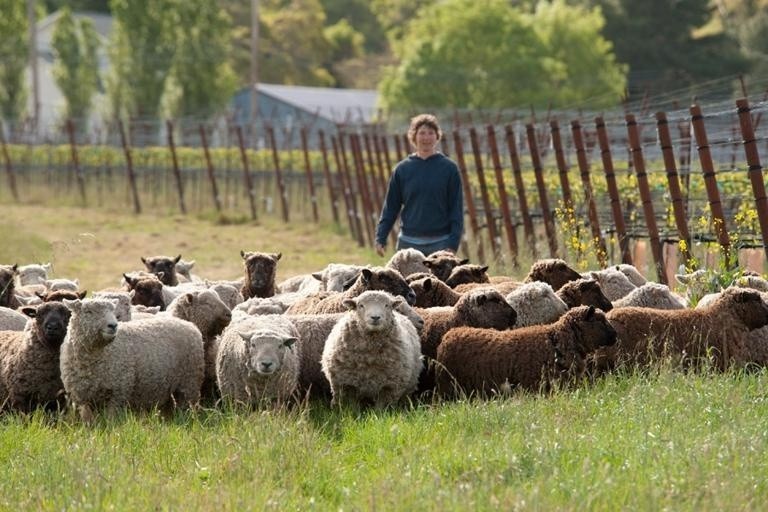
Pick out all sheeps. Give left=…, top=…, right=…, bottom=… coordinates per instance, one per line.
left=62, top=283, right=205, bottom=417
left=2, top=259, right=86, bottom=417
left=121, top=251, right=424, bottom=430
left=389, top=246, right=768, bottom=396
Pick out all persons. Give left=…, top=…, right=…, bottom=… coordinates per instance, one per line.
left=373, top=113, right=464, bottom=262
